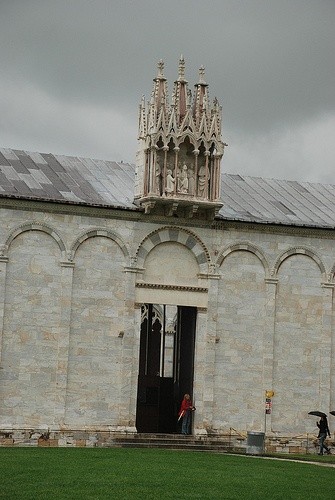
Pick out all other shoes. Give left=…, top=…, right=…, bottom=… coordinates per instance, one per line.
left=318, top=453, right=322, bottom=455
left=327, top=448, right=331, bottom=452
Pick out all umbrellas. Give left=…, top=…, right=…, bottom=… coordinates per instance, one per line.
left=308, top=411, right=327, bottom=417
left=329, top=411, right=335, bottom=416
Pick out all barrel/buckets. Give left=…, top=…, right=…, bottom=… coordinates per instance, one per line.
left=247, top=431, right=265, bottom=456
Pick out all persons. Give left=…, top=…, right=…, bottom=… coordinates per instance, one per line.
left=179, top=393, right=193, bottom=435
left=316, top=416, right=331, bottom=455
left=166, top=161, right=211, bottom=200
